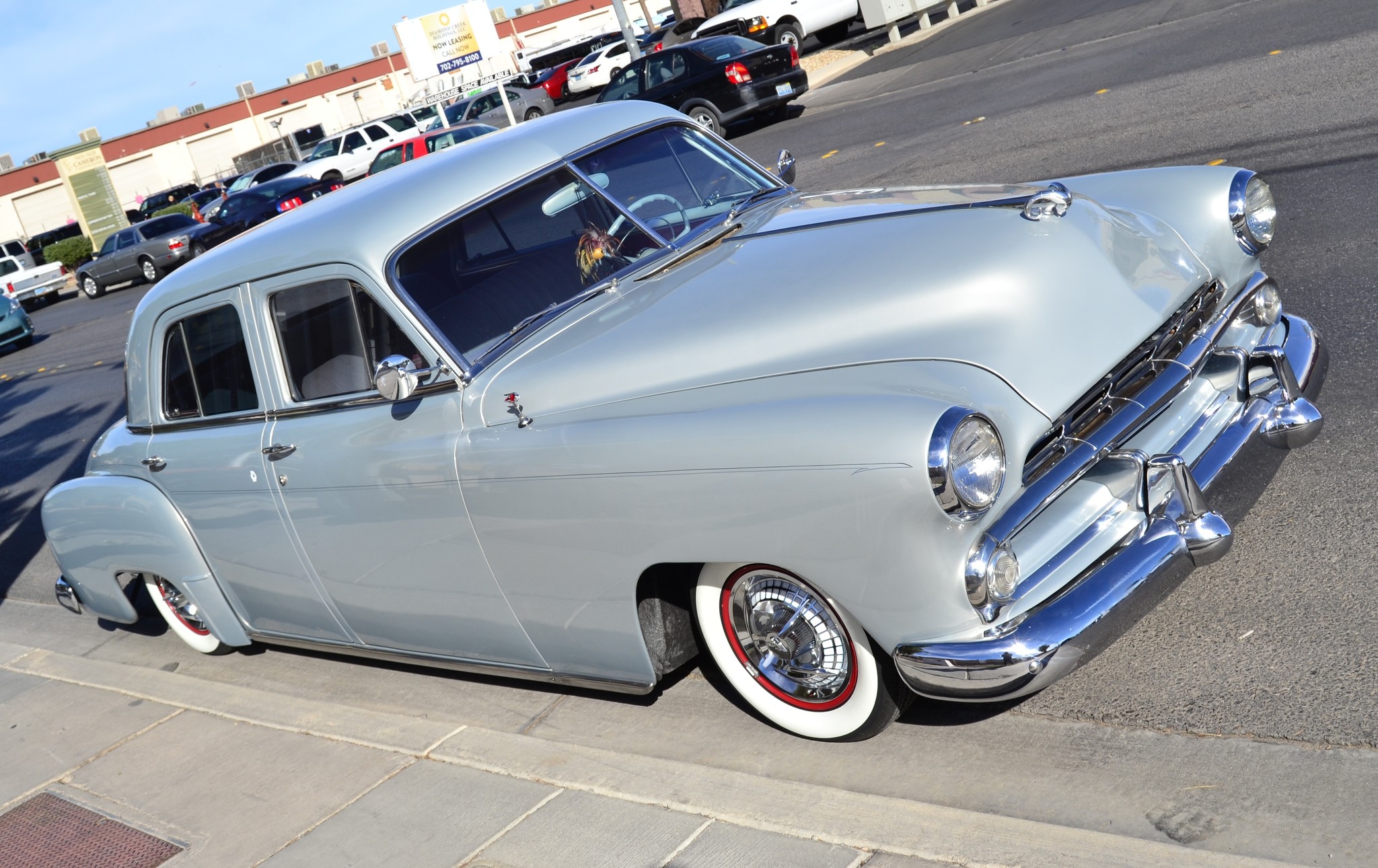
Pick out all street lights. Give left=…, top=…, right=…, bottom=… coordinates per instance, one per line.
left=270, top=117, right=294, bottom=160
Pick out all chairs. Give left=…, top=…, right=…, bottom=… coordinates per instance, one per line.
left=301, top=355, right=380, bottom=399
left=660, top=62, right=674, bottom=81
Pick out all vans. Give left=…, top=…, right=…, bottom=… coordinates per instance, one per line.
left=0, top=239, right=36, bottom=272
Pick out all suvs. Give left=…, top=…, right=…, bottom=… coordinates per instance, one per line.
left=300, top=113, right=423, bottom=186
left=132, top=182, right=199, bottom=222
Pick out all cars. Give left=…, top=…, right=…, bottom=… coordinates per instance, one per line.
left=199, top=173, right=245, bottom=191
left=38, top=97, right=1323, bottom=745
left=423, top=87, right=557, bottom=151
left=631, top=17, right=713, bottom=75
left=199, top=160, right=308, bottom=226
left=75, top=213, right=215, bottom=298
left=365, top=124, right=501, bottom=185
left=567, top=42, right=644, bottom=92
left=178, top=187, right=230, bottom=212
left=188, top=176, right=346, bottom=262
left=690, top=2, right=960, bottom=56
left=530, top=56, right=588, bottom=101
left=402, top=104, right=441, bottom=133
left=0, top=288, right=37, bottom=358
left=593, top=36, right=808, bottom=143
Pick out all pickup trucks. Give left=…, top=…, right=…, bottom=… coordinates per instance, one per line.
left=0, top=256, right=68, bottom=313
